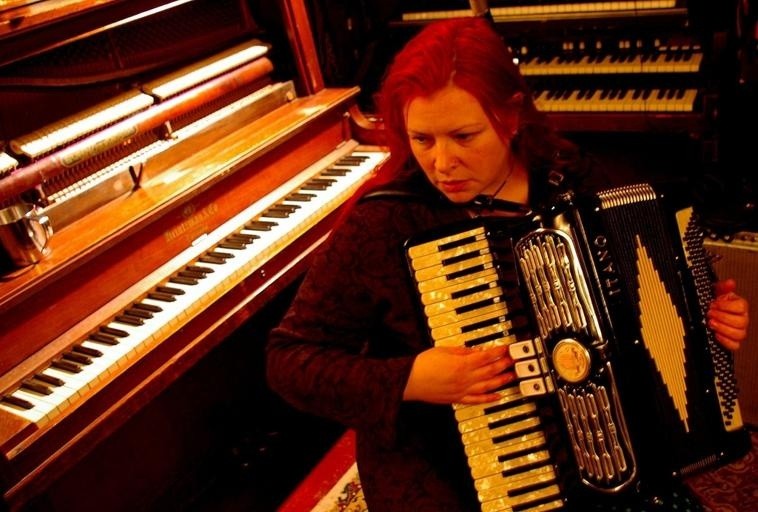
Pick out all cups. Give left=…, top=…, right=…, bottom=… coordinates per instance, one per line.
left=0, top=203, right=54, bottom=267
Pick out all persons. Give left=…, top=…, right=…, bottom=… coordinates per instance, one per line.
left=266, top=15, right=750, bottom=512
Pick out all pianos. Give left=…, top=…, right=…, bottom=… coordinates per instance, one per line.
left=0, top=0, right=392, bottom=512
left=402, top=1, right=746, bottom=135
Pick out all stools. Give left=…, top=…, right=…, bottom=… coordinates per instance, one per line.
left=277, top=428, right=370, bottom=511
